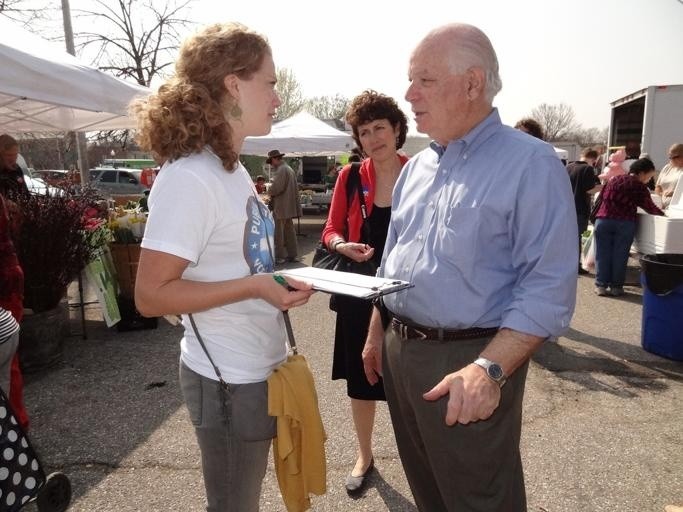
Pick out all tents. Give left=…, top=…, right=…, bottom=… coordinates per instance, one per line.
left=0, top=12, right=156, bottom=194
left=239, top=110, right=357, bottom=183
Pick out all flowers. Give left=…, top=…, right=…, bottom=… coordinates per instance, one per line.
left=2, top=164, right=117, bottom=315
left=107, top=203, right=150, bottom=244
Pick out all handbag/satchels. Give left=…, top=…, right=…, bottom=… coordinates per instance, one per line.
left=187, top=192, right=299, bottom=442
left=309, top=163, right=376, bottom=279
left=589, top=179, right=609, bottom=224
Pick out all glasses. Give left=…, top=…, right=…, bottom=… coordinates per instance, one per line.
left=669, top=155, right=681, bottom=160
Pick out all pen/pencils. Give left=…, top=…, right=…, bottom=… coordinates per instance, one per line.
left=271, top=271, right=295, bottom=292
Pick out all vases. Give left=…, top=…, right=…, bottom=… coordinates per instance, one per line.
left=16, top=297, right=70, bottom=376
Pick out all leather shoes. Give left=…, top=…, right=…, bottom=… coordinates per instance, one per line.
left=344, top=456, right=374, bottom=493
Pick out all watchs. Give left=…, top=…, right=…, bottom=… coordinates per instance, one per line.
left=474, top=357, right=508, bottom=388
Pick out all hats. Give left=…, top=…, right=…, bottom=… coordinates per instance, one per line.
left=266, top=150, right=285, bottom=163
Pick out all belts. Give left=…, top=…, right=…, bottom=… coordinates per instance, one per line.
left=388, top=316, right=500, bottom=342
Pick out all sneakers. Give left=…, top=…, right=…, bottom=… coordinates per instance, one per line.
left=609, top=286, right=625, bottom=296
left=593, top=285, right=607, bottom=297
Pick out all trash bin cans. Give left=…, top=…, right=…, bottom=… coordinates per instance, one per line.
left=639, top=248, right=683, bottom=362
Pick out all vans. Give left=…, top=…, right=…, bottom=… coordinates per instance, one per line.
left=88, top=169, right=156, bottom=193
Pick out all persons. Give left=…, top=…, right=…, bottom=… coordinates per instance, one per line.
left=566, top=146, right=604, bottom=276
left=654, top=143, right=683, bottom=199
left=0, top=134, right=28, bottom=199
left=265, top=150, right=303, bottom=265
left=129, top=22, right=317, bottom=512
left=347, top=155, right=360, bottom=166
left=513, top=118, right=544, bottom=141
left=351, top=148, right=365, bottom=162
left=324, top=165, right=338, bottom=214
left=0, top=307, right=20, bottom=400
left=254, top=175, right=268, bottom=195
left=639, top=153, right=655, bottom=191
left=593, top=157, right=669, bottom=297
left=321, top=90, right=412, bottom=493
left=0, top=194, right=30, bottom=434
left=362, top=20, right=577, bottom=512
